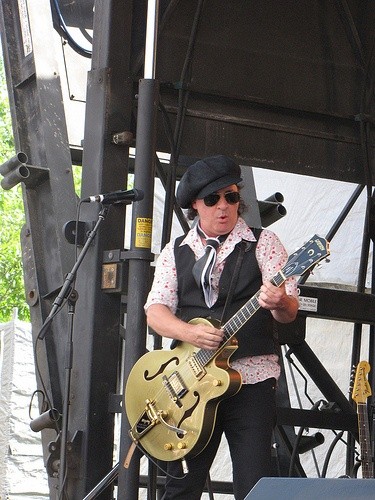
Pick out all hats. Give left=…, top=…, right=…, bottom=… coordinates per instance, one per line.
left=174, top=154, right=244, bottom=210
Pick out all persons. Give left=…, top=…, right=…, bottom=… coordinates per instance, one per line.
left=144, top=156, right=299, bottom=500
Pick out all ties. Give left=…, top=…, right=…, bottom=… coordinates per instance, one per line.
left=190, top=219, right=230, bottom=308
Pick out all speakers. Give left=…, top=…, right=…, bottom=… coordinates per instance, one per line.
left=244, top=477, right=375, bottom=500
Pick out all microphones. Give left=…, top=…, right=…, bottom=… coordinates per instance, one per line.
left=82, top=188, right=144, bottom=204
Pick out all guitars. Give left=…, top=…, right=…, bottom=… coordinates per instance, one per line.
left=124, top=233, right=332, bottom=463
left=351, top=360, right=375, bottom=479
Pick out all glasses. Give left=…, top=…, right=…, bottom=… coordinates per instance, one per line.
left=202, top=191, right=240, bottom=207
